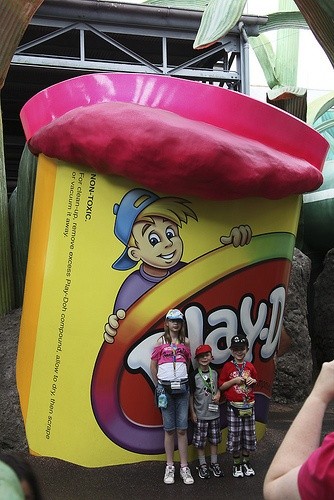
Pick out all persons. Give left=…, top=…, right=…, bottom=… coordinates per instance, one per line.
left=263, top=359, right=334, bottom=500
left=189, top=345, right=224, bottom=478
left=219, top=335, right=258, bottom=477
left=150, top=308, right=194, bottom=484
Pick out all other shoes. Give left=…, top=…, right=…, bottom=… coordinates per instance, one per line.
left=208, top=463, right=224, bottom=477
left=243, top=461, right=256, bottom=476
left=179, top=467, right=194, bottom=484
left=163, top=465, right=175, bottom=485
left=232, top=464, right=243, bottom=478
left=196, top=463, right=210, bottom=479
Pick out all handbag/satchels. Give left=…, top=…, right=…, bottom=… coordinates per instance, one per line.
left=230, top=400, right=255, bottom=417
left=162, top=380, right=189, bottom=395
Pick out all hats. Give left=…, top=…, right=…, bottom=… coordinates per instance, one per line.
left=166, top=309, right=183, bottom=320
left=229, top=335, right=249, bottom=349
left=194, top=345, right=212, bottom=359
